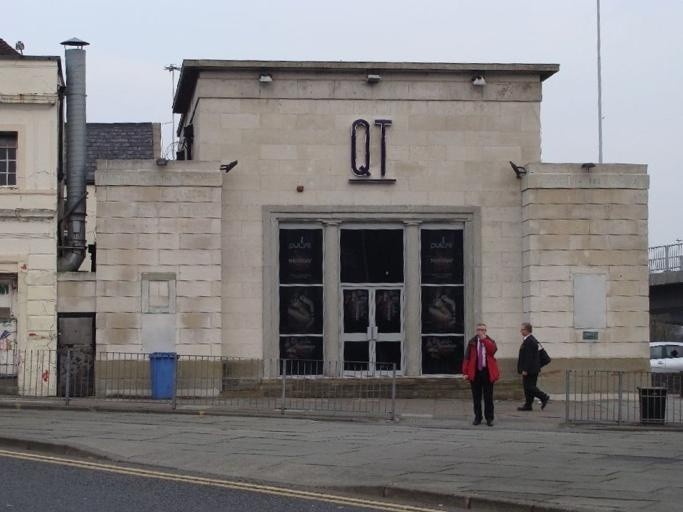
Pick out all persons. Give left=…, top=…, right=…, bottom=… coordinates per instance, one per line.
left=516, top=323, right=551, bottom=410
left=464, top=323, right=500, bottom=427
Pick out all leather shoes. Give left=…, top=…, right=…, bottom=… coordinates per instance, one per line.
left=472, top=415, right=483, bottom=425
left=541, top=395, right=550, bottom=409
left=487, top=420, right=494, bottom=427
left=517, top=406, right=533, bottom=411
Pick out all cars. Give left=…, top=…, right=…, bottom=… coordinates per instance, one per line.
left=649, top=341, right=683, bottom=393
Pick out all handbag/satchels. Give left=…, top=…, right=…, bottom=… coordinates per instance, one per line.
left=538, top=340, right=551, bottom=368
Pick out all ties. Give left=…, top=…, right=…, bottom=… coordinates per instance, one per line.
left=478, top=340, right=482, bottom=371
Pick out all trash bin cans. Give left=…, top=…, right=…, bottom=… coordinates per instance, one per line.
left=149, top=352, right=177, bottom=400
left=636, top=387, right=667, bottom=424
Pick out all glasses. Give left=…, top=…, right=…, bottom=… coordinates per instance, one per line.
left=477, top=329, right=486, bottom=331
left=521, top=329, right=529, bottom=331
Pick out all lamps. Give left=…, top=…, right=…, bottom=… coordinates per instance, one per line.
left=220, top=160, right=238, bottom=173
left=581, top=163, right=596, bottom=170
left=472, top=73, right=488, bottom=87
left=258, top=71, right=273, bottom=84
left=157, top=158, right=168, bottom=166
left=510, top=161, right=526, bottom=177
left=367, top=70, right=383, bottom=83
